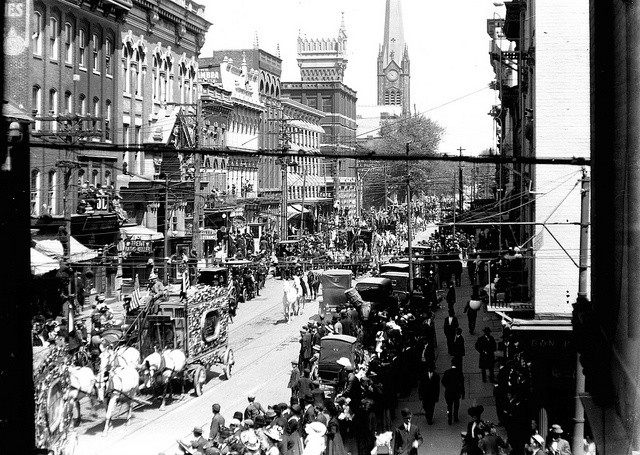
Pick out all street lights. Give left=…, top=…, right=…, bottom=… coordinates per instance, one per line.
left=59, top=183, right=98, bottom=344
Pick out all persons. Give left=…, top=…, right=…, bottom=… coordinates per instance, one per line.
left=502, top=385, right=530, bottom=448
left=418, top=361, right=442, bottom=424
left=446, top=281, right=460, bottom=314
left=476, top=327, right=497, bottom=382
left=393, top=407, right=421, bottom=454
left=584, top=432, right=596, bottom=453
left=518, top=351, right=528, bottom=368
left=530, top=420, right=541, bottom=434
left=374, top=300, right=439, bottom=390
left=176, top=396, right=362, bottom=454
left=464, top=295, right=482, bottom=332
left=461, top=406, right=492, bottom=454
left=549, top=424, right=569, bottom=455
left=440, top=357, right=464, bottom=426
left=416, top=362, right=436, bottom=372
left=288, top=303, right=358, bottom=404
left=370, top=414, right=393, bottom=454
left=475, top=421, right=507, bottom=454
left=443, top=309, right=458, bottom=353
left=525, top=434, right=543, bottom=454
left=450, top=328, right=465, bottom=368
left=492, top=355, right=509, bottom=426
left=500, top=333, right=516, bottom=362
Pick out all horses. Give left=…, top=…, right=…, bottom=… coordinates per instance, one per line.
left=293, top=278, right=310, bottom=312
left=308, top=272, right=321, bottom=300
left=98, top=345, right=140, bottom=373
left=281, top=280, right=298, bottom=323
left=92, top=367, right=140, bottom=437
left=293, top=275, right=307, bottom=285
left=138, top=350, right=188, bottom=410
left=53, top=366, right=95, bottom=428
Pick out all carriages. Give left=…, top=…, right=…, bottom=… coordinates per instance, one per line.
left=69, top=285, right=234, bottom=437
left=347, top=230, right=372, bottom=281
left=33, top=346, right=69, bottom=455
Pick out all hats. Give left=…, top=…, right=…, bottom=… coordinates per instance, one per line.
left=233, top=411, right=242, bottom=420
left=291, top=405, right=301, bottom=412
left=264, top=424, right=283, bottom=442
left=402, top=409, right=412, bottom=416
left=177, top=438, right=195, bottom=455
left=213, top=404, right=220, bottom=412
left=304, top=369, right=310, bottom=373
left=291, top=360, right=298, bottom=364
left=549, top=424, right=564, bottom=433
left=306, top=422, right=326, bottom=437
left=428, top=362, right=436, bottom=369
left=532, top=435, right=544, bottom=446
left=337, top=357, right=351, bottom=367
left=193, top=426, right=204, bottom=433
left=341, top=312, right=347, bottom=316
left=290, top=397, right=298, bottom=404
left=254, top=416, right=265, bottom=424
left=266, top=410, right=276, bottom=418
left=482, top=327, right=492, bottom=332
left=240, top=429, right=260, bottom=451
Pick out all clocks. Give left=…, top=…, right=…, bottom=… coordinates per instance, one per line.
left=386, top=69, right=400, bottom=82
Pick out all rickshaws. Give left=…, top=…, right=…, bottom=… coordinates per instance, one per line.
left=352, top=278, right=391, bottom=323
left=379, top=264, right=409, bottom=276
left=404, top=247, right=433, bottom=281
left=307, top=335, right=358, bottom=416
left=224, top=260, right=254, bottom=302
left=277, top=239, right=302, bottom=279
left=195, top=268, right=233, bottom=316
left=319, top=269, right=351, bottom=321
left=398, top=258, right=424, bottom=293
left=380, top=272, right=409, bottom=310
